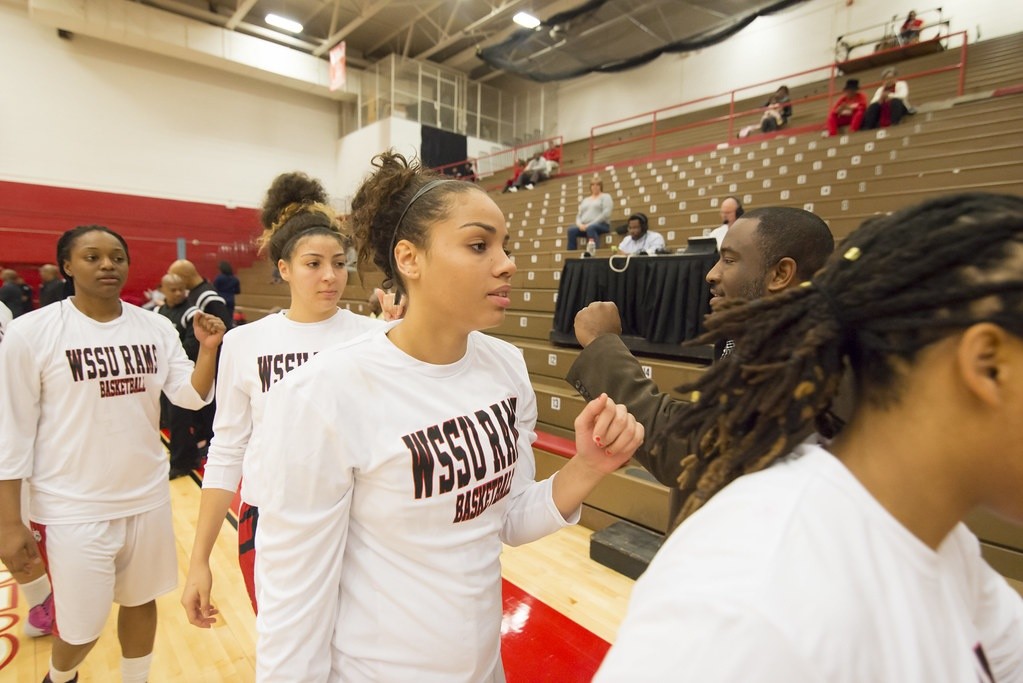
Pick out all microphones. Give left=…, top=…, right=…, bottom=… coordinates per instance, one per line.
left=723, top=220, right=728, bottom=224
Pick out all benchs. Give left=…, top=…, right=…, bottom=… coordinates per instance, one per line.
left=212, top=32, right=1023, bottom=584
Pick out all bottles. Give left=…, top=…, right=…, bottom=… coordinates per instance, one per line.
left=586, top=238, right=596, bottom=258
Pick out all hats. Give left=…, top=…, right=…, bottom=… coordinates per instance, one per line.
left=843, top=79, right=860, bottom=91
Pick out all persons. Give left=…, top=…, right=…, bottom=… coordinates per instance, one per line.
left=755, top=86, right=792, bottom=132
left=861, top=66, right=916, bottom=131
left=563, top=203, right=833, bottom=528
left=901, top=10, right=923, bottom=44
left=566, top=178, right=614, bottom=257
left=827, top=79, right=868, bottom=137
left=0, top=259, right=240, bottom=480
left=593, top=190, right=1023, bottom=683
left=618, top=212, right=667, bottom=254
left=176, top=171, right=387, bottom=629
left=0, top=224, right=226, bottom=683
left=240, top=146, right=646, bottom=683
left=707, top=196, right=745, bottom=251
left=448, top=162, right=475, bottom=183
left=501, top=140, right=560, bottom=194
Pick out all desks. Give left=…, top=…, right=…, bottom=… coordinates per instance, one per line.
left=549, top=252, right=718, bottom=361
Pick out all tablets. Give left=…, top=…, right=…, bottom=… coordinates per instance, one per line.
left=687, top=237, right=717, bottom=245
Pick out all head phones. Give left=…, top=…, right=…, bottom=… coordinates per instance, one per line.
left=727, top=196, right=744, bottom=218
left=635, top=214, right=648, bottom=232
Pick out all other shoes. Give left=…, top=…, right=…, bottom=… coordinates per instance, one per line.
left=41, top=670, right=78, bottom=683
left=25, top=592, right=57, bottom=638
left=508, top=187, right=518, bottom=193
left=524, top=183, right=534, bottom=190
